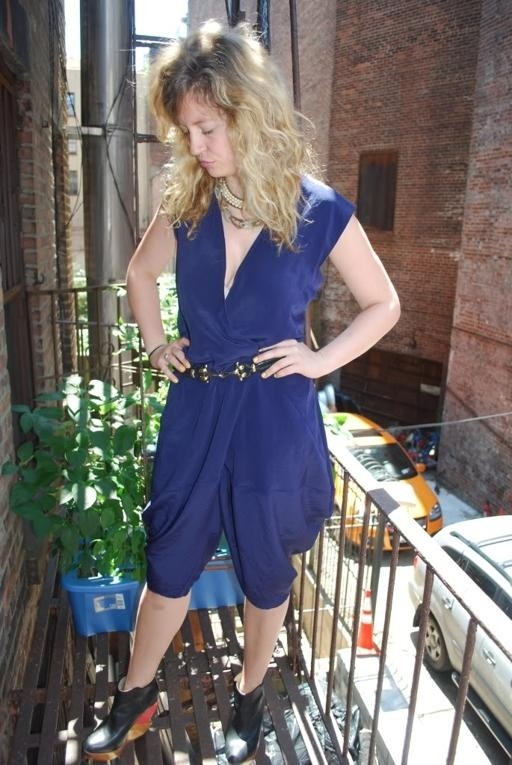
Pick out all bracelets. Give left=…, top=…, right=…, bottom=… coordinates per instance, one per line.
left=149, top=344, right=165, bottom=359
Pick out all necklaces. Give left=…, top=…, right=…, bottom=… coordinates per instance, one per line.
left=219, top=196, right=264, bottom=229
left=219, top=177, right=244, bottom=210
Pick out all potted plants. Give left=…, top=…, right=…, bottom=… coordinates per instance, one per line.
left=2, top=370, right=244, bottom=638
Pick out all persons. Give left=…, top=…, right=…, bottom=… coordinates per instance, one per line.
left=83, top=18, right=400, bottom=762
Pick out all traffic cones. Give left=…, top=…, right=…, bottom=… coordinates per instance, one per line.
left=354, top=589, right=382, bottom=658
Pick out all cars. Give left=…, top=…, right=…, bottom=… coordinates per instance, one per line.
left=406, top=516, right=512, bottom=762
left=322, top=410, right=445, bottom=553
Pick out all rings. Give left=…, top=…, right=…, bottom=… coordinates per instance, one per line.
left=163, top=352, right=168, bottom=360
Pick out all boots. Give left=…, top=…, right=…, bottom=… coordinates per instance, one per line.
left=224, top=670, right=265, bottom=765
left=83, top=674, right=159, bottom=761
